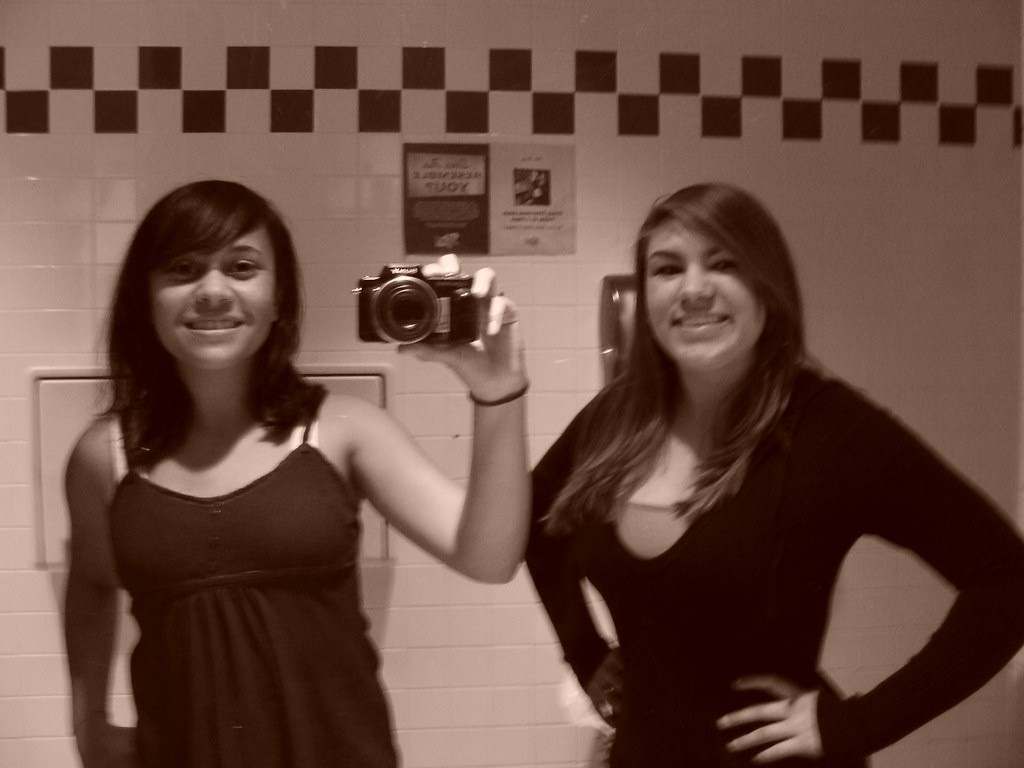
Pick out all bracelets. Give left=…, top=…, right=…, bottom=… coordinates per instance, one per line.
left=470, top=380, right=529, bottom=407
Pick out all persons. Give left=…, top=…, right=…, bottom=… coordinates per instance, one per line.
left=525, top=185, right=1024, bottom=768
left=62, top=180, right=531, bottom=767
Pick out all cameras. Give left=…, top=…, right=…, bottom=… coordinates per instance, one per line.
left=355, top=263, right=482, bottom=347
